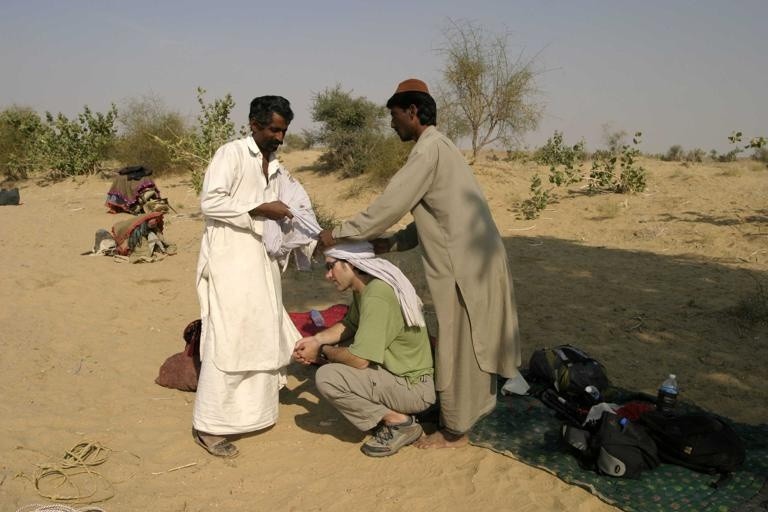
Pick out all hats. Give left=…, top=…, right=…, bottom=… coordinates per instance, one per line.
left=396, top=78, right=429, bottom=94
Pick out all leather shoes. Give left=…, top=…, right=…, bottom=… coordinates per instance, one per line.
left=192, top=425, right=240, bottom=458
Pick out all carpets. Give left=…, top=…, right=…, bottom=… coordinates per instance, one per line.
left=290, top=304, right=436, bottom=368
left=462, top=367, right=768, bottom=511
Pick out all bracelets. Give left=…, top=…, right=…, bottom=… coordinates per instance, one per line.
left=318, top=342, right=329, bottom=362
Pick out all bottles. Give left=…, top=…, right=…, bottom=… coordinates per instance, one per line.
left=658, top=373, right=679, bottom=410
left=310, top=308, right=324, bottom=327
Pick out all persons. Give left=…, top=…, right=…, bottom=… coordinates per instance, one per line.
left=189, top=93, right=324, bottom=460
left=289, top=238, right=438, bottom=460
left=313, top=76, right=522, bottom=440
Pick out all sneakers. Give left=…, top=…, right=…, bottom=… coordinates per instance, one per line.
left=360, top=415, right=423, bottom=457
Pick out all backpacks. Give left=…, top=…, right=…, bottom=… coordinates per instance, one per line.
left=631, top=397, right=746, bottom=473
left=533, top=342, right=619, bottom=400
left=560, top=411, right=661, bottom=477
